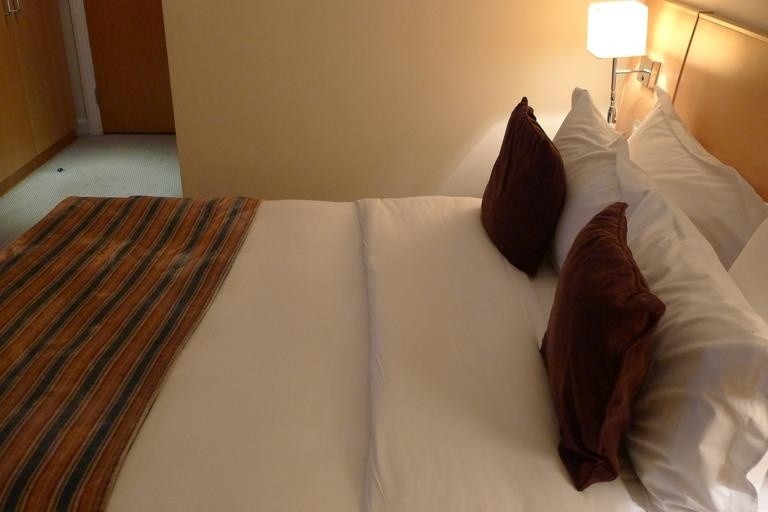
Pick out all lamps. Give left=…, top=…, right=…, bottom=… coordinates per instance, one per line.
left=585, top=0, right=661, bottom=124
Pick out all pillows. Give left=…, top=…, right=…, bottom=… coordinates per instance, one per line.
left=550, top=86, right=620, bottom=277
left=727, top=206, right=768, bottom=320
left=478, top=96, right=569, bottom=279
left=539, top=202, right=665, bottom=492
left=624, top=184, right=768, bottom=512
left=625, top=84, right=768, bottom=266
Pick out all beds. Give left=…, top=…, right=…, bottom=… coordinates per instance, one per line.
left=0, top=193, right=768, bottom=512
